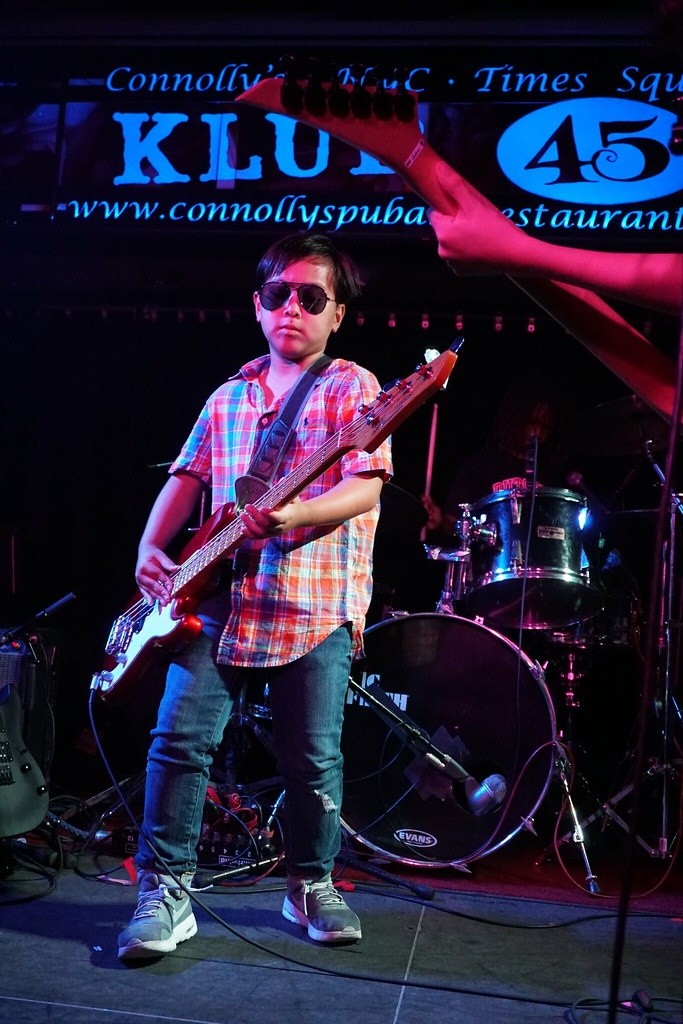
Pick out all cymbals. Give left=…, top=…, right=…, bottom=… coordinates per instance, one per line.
left=565, top=393, right=683, bottom=457
left=609, top=508, right=671, bottom=521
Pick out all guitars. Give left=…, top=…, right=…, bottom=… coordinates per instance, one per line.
left=232, top=53, right=683, bottom=436
left=91, top=338, right=464, bottom=701
left=1, top=682, right=50, bottom=840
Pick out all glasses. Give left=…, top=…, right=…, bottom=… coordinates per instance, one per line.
left=257, top=281, right=339, bottom=315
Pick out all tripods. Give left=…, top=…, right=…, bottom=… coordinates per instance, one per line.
left=55, top=682, right=280, bottom=834
left=554, top=423, right=683, bottom=901
left=198, top=677, right=479, bottom=904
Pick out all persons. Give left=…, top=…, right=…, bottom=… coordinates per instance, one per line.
left=420, top=379, right=584, bottom=539
left=117, top=231, right=395, bottom=960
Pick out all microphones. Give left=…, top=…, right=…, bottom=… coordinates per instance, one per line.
left=567, top=472, right=610, bottom=516
left=525, top=435, right=536, bottom=477
left=466, top=774, right=510, bottom=818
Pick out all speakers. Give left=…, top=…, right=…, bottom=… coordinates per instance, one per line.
left=0, top=628, right=60, bottom=785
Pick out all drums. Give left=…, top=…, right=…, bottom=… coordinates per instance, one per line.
left=546, top=585, right=643, bottom=652
left=314, top=609, right=558, bottom=871
left=445, top=488, right=608, bottom=631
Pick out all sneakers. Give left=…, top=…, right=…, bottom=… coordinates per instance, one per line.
left=118, top=872, right=197, bottom=961
left=282, top=871, right=362, bottom=941
